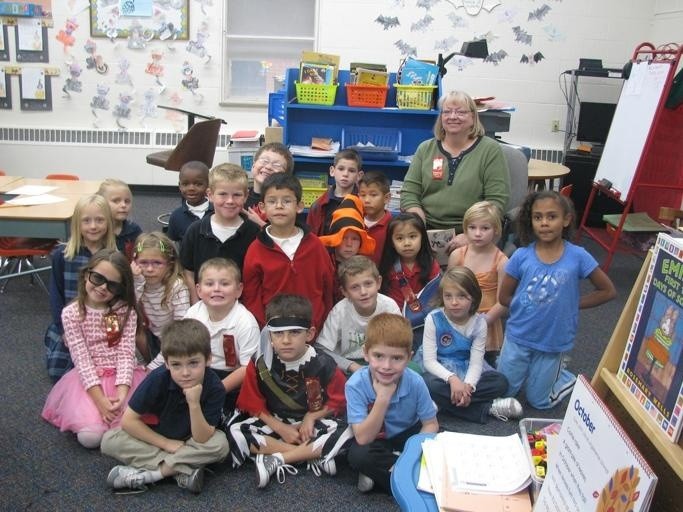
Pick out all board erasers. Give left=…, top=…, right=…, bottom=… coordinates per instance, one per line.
left=599, top=178, right=612, bottom=189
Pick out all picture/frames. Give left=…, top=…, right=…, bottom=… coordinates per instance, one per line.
left=87, top=0, right=190, bottom=41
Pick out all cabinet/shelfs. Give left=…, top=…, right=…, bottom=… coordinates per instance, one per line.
left=281, top=65, right=441, bottom=218
left=584, top=250, right=682, bottom=512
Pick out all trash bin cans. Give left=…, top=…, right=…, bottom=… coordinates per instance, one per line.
left=225, top=131, right=265, bottom=188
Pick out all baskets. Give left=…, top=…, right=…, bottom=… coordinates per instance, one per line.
left=293, top=77, right=341, bottom=105
left=343, top=79, right=391, bottom=108
left=393, top=80, right=440, bottom=111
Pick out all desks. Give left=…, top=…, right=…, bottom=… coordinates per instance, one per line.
left=523, top=154, right=571, bottom=191
left=0, top=174, right=106, bottom=243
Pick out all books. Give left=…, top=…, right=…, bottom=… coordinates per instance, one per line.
left=298, top=52, right=439, bottom=107
left=616, top=232, right=683, bottom=443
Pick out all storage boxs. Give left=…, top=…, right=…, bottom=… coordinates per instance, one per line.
left=515, top=414, right=564, bottom=502
left=225, top=140, right=264, bottom=179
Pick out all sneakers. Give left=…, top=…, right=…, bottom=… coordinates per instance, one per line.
left=306, top=454, right=338, bottom=476
left=105, top=463, right=144, bottom=492
left=253, top=451, right=280, bottom=488
left=173, top=467, right=205, bottom=494
left=488, top=396, right=524, bottom=421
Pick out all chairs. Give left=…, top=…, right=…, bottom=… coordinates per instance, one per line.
left=143, top=116, right=221, bottom=231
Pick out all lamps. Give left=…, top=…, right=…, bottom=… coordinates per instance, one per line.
left=437, top=35, right=487, bottom=79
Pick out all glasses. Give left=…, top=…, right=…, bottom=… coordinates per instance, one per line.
left=84, top=269, right=122, bottom=297
left=254, top=157, right=286, bottom=174
left=441, top=107, right=472, bottom=116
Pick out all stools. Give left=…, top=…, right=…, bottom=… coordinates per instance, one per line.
left=0, top=237, right=61, bottom=295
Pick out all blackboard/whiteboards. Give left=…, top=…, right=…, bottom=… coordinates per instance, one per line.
left=593, top=59, right=674, bottom=206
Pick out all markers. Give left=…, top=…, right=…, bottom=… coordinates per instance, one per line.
left=609, top=187, right=620, bottom=197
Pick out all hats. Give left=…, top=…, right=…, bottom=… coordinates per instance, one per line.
left=317, top=193, right=376, bottom=257
left=265, top=314, right=311, bottom=333
